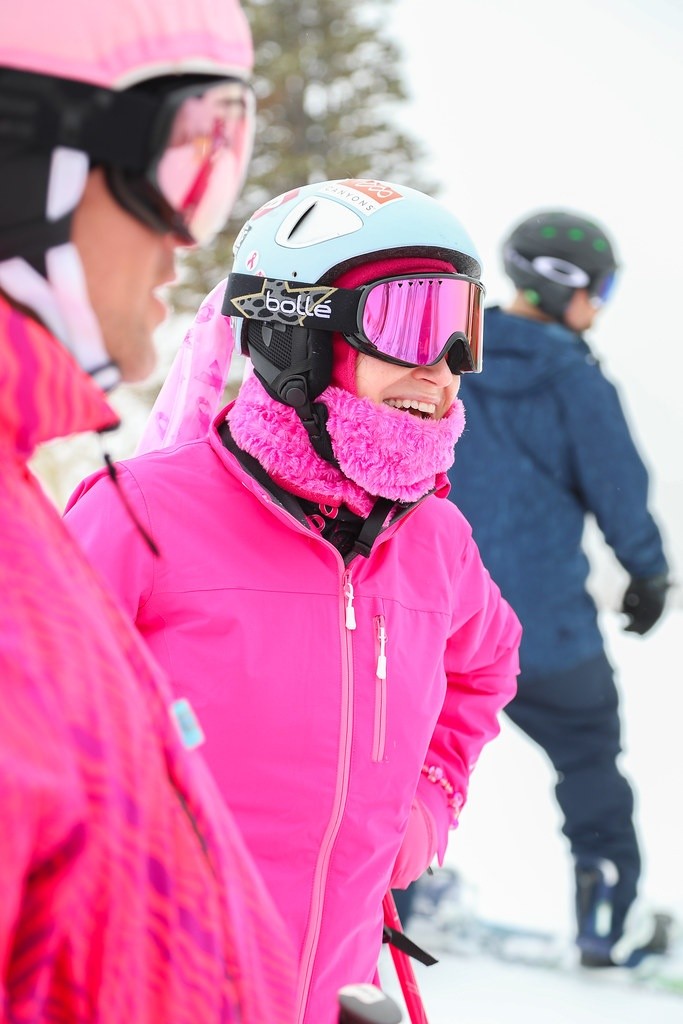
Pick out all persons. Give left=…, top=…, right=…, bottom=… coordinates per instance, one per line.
left=391, top=212, right=670, bottom=965
left=62, top=178, right=524, bottom=1024
left=0, top=1, right=299, bottom=1024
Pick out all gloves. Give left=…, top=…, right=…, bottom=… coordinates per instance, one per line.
left=617, top=576, right=667, bottom=635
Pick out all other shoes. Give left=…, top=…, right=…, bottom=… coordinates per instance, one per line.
left=577, top=911, right=672, bottom=969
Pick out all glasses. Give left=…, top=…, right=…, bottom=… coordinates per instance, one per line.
left=344, top=272, right=485, bottom=375
left=588, top=270, right=619, bottom=303
left=106, top=78, right=256, bottom=249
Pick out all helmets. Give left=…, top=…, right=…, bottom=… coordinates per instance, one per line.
left=233, top=178, right=484, bottom=407
left=503, top=211, right=616, bottom=315
left=0, top=0, right=254, bottom=92
